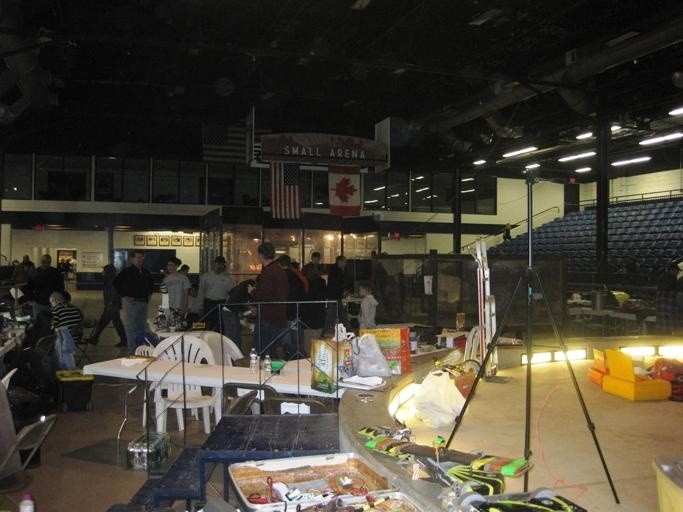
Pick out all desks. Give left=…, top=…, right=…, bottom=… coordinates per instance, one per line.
left=83, top=357, right=346, bottom=433
left=565, top=307, right=656, bottom=338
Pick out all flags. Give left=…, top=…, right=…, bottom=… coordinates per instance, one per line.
left=271, top=162, right=301, bottom=220
left=327, top=166, right=361, bottom=217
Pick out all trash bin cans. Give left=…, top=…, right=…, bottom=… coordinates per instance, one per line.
left=56, top=370, right=95, bottom=412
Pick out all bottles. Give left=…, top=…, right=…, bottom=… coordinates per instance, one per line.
left=263, top=354, right=272, bottom=382
left=20, top=494, right=34, bottom=512
left=249, top=348, right=258, bottom=374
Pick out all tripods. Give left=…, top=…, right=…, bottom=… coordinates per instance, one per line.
left=443, top=265, right=622, bottom=505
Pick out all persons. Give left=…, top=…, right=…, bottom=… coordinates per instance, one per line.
left=1, top=254, right=82, bottom=372
left=84, top=264, right=127, bottom=346
left=249, top=240, right=380, bottom=359
left=160, top=257, right=256, bottom=349
left=112, top=249, right=154, bottom=358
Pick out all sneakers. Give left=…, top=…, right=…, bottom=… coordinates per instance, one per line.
left=84, top=336, right=98, bottom=344
left=116, top=343, right=128, bottom=347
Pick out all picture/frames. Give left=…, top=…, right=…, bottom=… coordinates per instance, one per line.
left=133, top=235, right=201, bottom=246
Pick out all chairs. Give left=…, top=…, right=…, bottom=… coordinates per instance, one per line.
left=153, top=335, right=220, bottom=433
left=487, top=200, right=683, bottom=273
left=133, top=345, right=151, bottom=428
left=0, top=414, right=57, bottom=512
left=203, top=332, right=245, bottom=366
